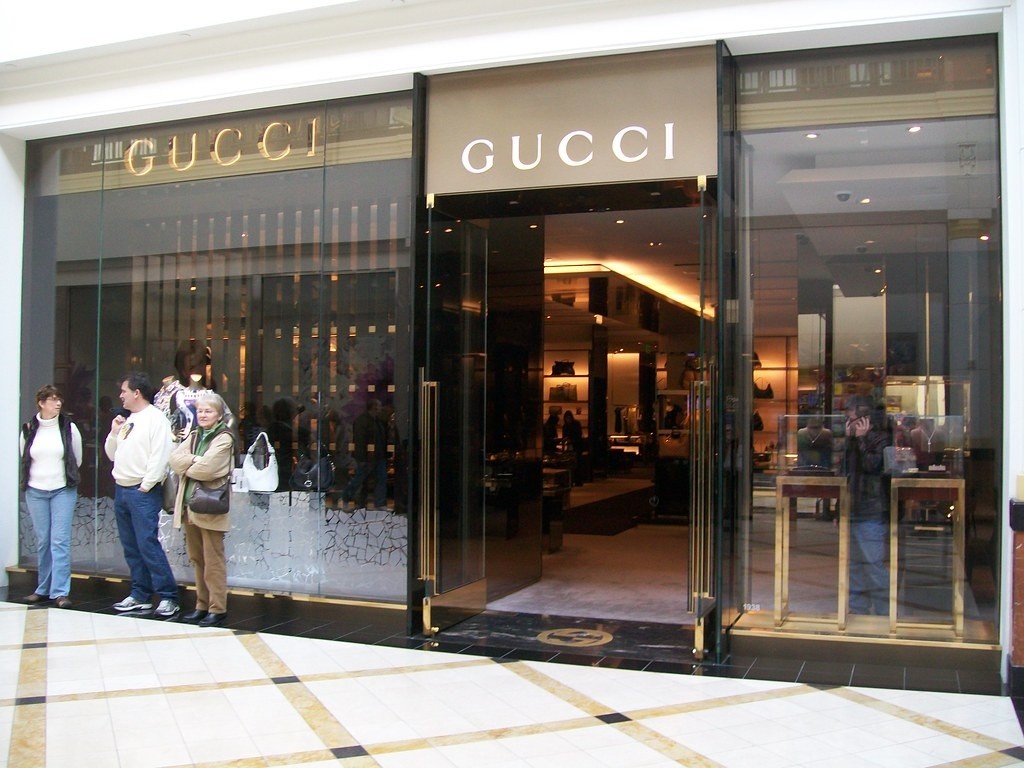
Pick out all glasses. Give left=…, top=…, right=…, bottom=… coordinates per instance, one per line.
left=845, top=414, right=866, bottom=425
left=41, top=396, right=64, bottom=404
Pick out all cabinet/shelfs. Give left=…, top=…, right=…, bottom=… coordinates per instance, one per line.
left=752, top=366, right=799, bottom=481
left=541, top=373, right=589, bottom=405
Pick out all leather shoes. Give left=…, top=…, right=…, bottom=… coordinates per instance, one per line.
left=180, top=609, right=209, bottom=623
left=198, top=612, right=228, bottom=628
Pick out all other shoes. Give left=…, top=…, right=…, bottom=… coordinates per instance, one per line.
left=23, top=593, right=47, bottom=602
left=55, top=596, right=71, bottom=608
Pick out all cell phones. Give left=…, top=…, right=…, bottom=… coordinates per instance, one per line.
left=847, top=415, right=875, bottom=437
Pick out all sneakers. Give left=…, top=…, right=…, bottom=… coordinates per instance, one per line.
left=112, top=594, right=153, bottom=611
left=154, top=598, right=181, bottom=616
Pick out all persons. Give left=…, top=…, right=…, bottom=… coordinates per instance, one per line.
left=169, top=393, right=238, bottom=628
left=153, top=373, right=235, bottom=492
left=909, top=419, right=944, bottom=466
left=19, top=382, right=82, bottom=608
left=543, top=411, right=584, bottom=488
left=344, top=335, right=394, bottom=511
left=796, top=409, right=832, bottom=468
left=841, top=394, right=899, bottom=617
left=105, top=372, right=179, bottom=616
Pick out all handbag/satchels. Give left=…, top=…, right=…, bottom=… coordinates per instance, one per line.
left=243, top=432, right=280, bottom=494
left=753, top=378, right=774, bottom=399
left=753, top=408, right=763, bottom=430
left=188, top=429, right=234, bottom=514
left=551, top=359, right=575, bottom=376
left=550, top=383, right=577, bottom=402
left=288, top=441, right=335, bottom=508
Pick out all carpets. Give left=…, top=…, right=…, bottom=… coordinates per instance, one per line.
left=562, top=485, right=654, bottom=537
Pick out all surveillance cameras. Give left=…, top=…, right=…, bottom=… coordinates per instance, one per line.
left=835, top=191, right=852, bottom=201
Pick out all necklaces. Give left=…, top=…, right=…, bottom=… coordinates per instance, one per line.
left=922, top=428, right=935, bottom=453
left=805, top=427, right=823, bottom=445
